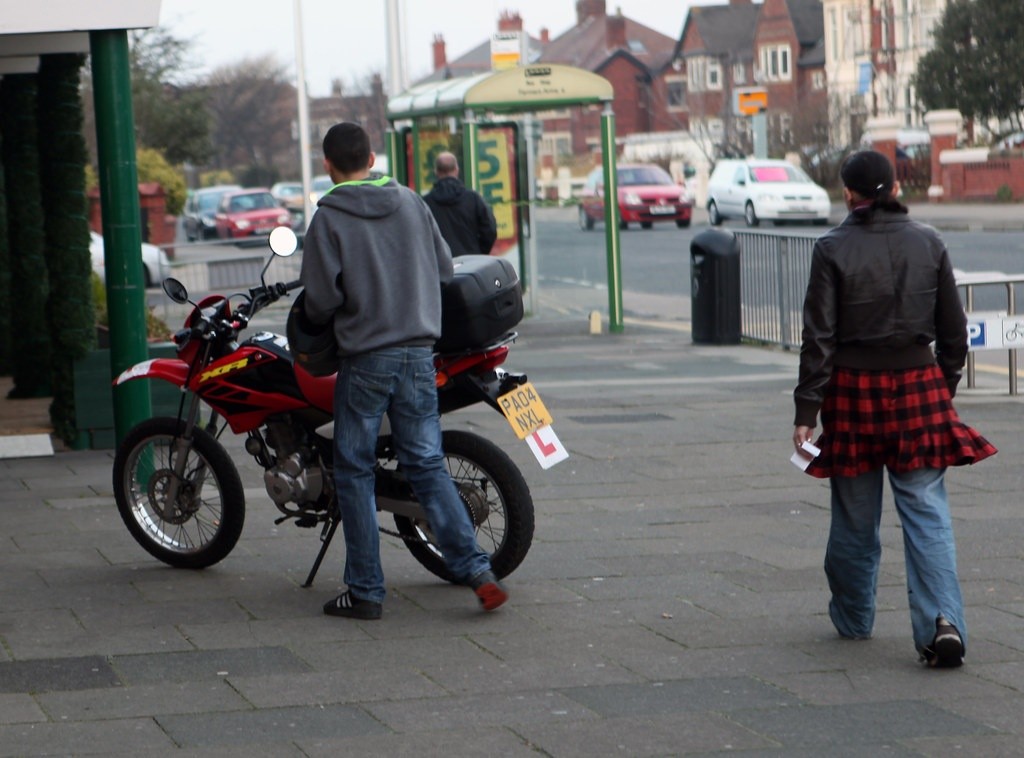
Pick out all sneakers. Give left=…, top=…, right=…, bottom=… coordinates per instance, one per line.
left=927, top=613, right=965, bottom=669
left=323, top=591, right=383, bottom=620
left=469, top=569, right=509, bottom=611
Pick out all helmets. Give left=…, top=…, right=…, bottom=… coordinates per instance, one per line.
left=286, top=287, right=338, bottom=377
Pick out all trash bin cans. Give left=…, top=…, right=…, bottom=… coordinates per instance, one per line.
left=685, top=225, right=742, bottom=346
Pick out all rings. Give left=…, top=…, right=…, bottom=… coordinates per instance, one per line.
left=797, top=442, right=801, bottom=446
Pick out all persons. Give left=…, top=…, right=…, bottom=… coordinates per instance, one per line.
left=794, top=150, right=997, bottom=668
left=422, top=153, right=497, bottom=255
left=302, top=123, right=508, bottom=620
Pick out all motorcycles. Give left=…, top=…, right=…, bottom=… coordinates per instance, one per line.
left=111, top=227, right=570, bottom=587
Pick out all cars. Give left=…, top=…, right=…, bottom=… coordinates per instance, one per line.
left=181, top=172, right=334, bottom=241
left=577, top=161, right=696, bottom=231
left=705, top=158, right=831, bottom=228
left=87, top=230, right=172, bottom=288
